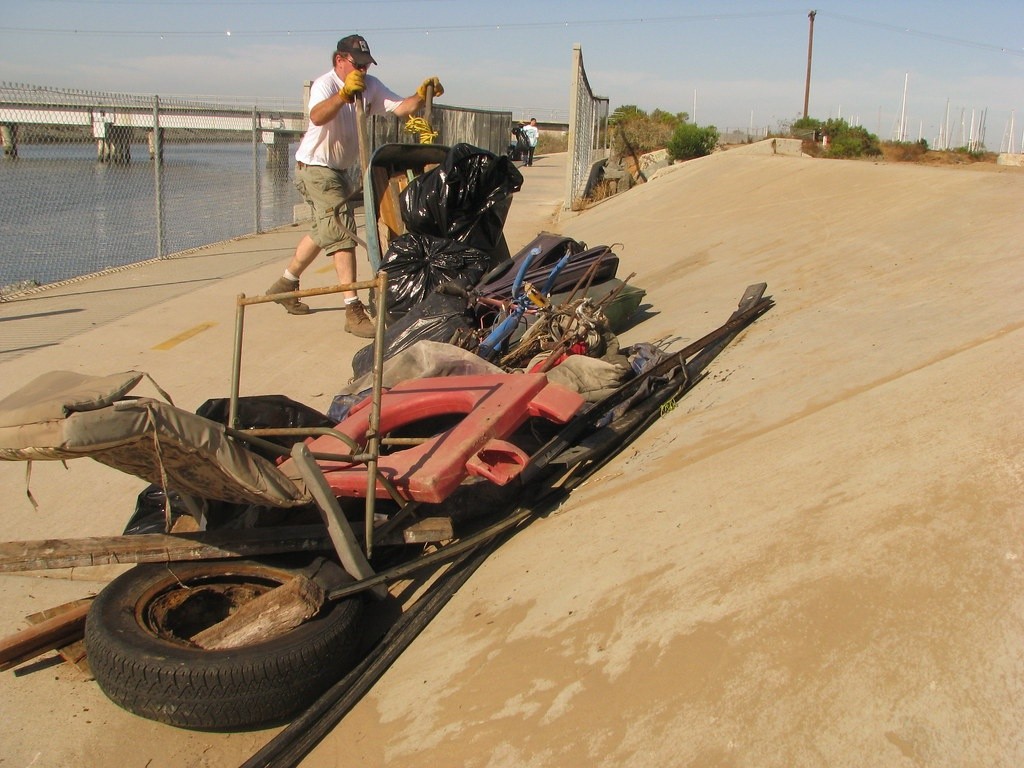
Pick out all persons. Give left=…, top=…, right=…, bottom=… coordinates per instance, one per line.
left=265, top=34, right=444, bottom=338
left=523, top=118, right=539, bottom=167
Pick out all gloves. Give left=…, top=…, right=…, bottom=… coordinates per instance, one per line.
left=418, top=77, right=444, bottom=101
left=339, top=70, right=365, bottom=103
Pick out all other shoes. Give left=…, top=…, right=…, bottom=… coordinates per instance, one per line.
left=522, top=163, right=527, bottom=166
left=528, top=164, right=531, bottom=167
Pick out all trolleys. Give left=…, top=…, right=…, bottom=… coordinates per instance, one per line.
left=351, top=73, right=457, bottom=325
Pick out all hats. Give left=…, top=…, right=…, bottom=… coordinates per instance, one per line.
left=337, top=34, right=378, bottom=66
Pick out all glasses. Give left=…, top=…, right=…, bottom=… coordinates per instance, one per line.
left=341, top=55, right=370, bottom=70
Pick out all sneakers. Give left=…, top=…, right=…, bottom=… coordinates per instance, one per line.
left=265, top=276, right=309, bottom=314
left=344, top=301, right=376, bottom=338
